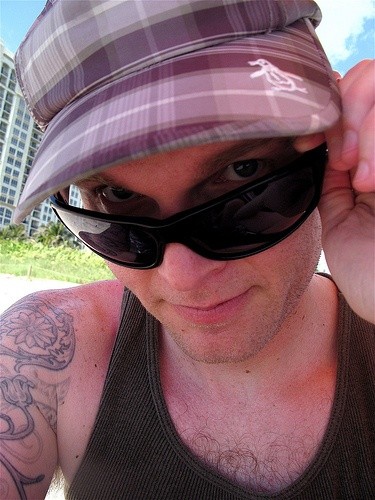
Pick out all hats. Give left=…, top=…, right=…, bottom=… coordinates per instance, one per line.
left=12, top=0, right=344, bottom=228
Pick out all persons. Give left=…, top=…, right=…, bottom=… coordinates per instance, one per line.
left=0, top=0, right=375, bottom=500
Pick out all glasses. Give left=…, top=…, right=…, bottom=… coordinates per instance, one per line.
left=49, top=144, right=328, bottom=270
